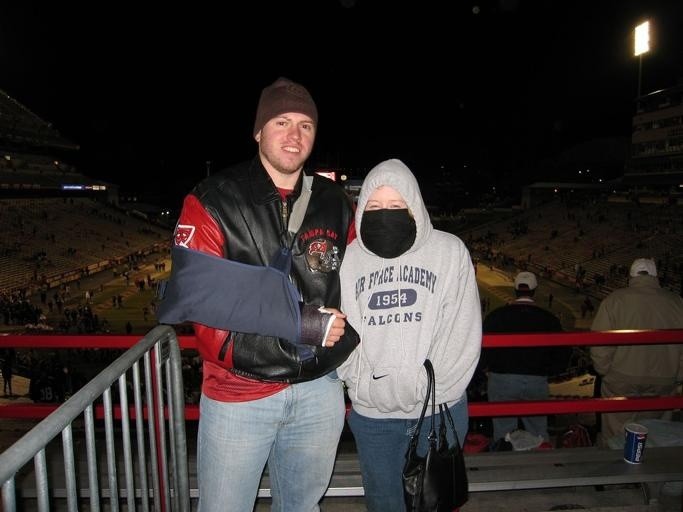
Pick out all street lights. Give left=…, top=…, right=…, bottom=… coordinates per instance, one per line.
left=632, top=19, right=652, bottom=112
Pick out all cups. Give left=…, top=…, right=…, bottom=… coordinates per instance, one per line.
left=624, top=424, right=649, bottom=466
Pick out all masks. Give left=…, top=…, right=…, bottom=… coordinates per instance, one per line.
left=359, top=206, right=409, bottom=239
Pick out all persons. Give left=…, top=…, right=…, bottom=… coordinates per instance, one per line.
left=158, top=75, right=355, bottom=512
left=587, top=257, right=683, bottom=439
left=1, top=180, right=202, bottom=414
left=476, top=270, right=573, bottom=444
left=335, top=156, right=486, bottom=512
left=470, top=206, right=683, bottom=318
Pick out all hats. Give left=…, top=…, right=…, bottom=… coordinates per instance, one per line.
left=251, top=77, right=320, bottom=137
left=512, top=269, right=537, bottom=296
left=628, top=256, right=659, bottom=280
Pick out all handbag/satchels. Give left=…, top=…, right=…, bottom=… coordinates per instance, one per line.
left=400, top=358, right=468, bottom=510
